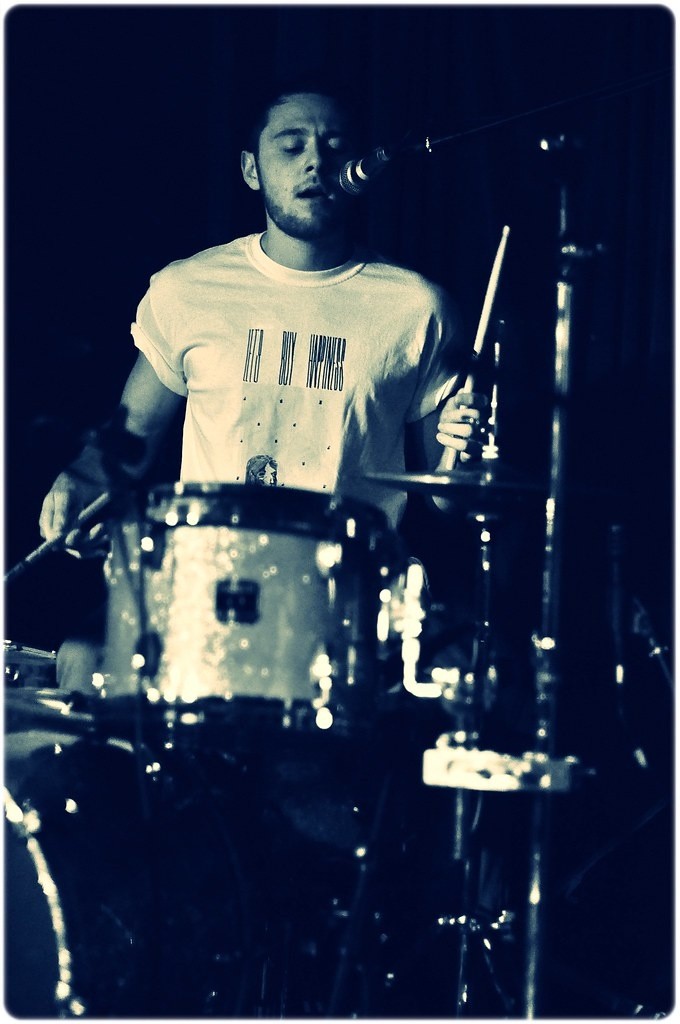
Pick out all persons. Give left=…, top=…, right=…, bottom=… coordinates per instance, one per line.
left=40, top=79, right=488, bottom=548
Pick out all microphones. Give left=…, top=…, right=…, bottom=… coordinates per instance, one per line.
left=338, top=148, right=394, bottom=195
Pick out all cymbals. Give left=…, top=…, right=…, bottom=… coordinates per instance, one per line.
left=361, top=465, right=552, bottom=501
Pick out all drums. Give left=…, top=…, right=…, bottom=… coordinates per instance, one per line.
left=100, top=480, right=389, bottom=716
